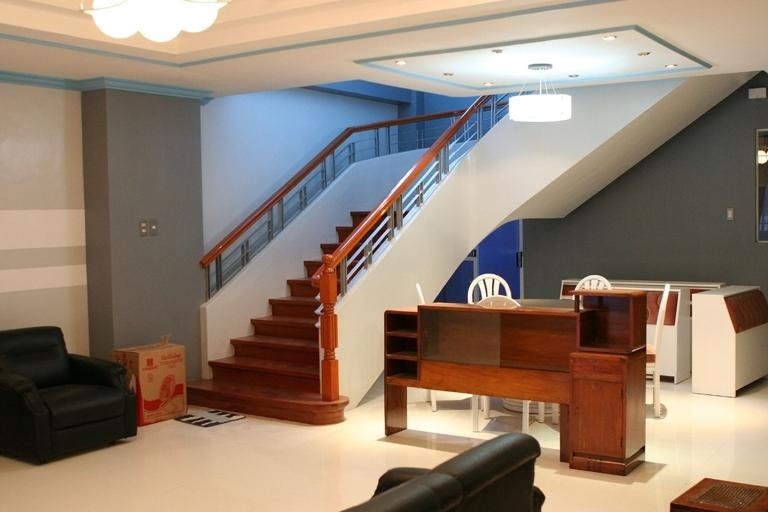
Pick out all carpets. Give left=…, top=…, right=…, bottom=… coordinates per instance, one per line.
left=174, top=409, right=246, bottom=427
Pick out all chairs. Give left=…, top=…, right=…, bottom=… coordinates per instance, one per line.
left=340, top=431, right=545, bottom=511
left=0, top=326, right=137, bottom=466
left=415, top=274, right=670, bottom=434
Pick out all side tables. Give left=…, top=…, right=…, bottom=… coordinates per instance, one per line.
left=670, top=478, right=768, bottom=512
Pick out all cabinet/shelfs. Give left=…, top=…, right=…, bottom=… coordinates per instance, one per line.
left=384, top=289, right=647, bottom=477
left=560, top=279, right=768, bottom=399
left=437, top=219, right=524, bottom=301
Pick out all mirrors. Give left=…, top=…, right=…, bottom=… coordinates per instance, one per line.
left=755, top=129, right=768, bottom=246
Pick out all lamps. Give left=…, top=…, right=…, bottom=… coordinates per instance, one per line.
left=80, top=0, right=230, bottom=45
left=508, top=64, right=572, bottom=124
left=758, top=136, right=768, bottom=164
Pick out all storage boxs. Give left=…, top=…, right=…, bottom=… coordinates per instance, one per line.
left=112, top=342, right=188, bottom=427
